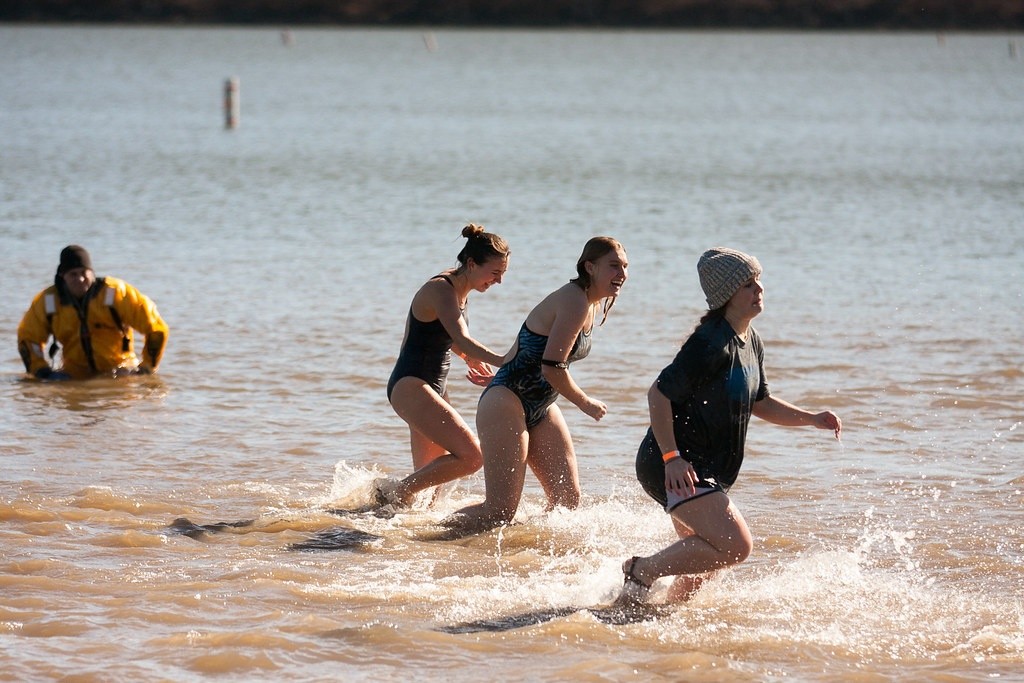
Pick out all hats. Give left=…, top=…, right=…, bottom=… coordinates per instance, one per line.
left=60, top=244, right=93, bottom=274
left=697, top=248, right=762, bottom=310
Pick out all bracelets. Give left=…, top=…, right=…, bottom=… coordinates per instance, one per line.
left=459, top=353, right=466, bottom=359
left=662, top=450, right=681, bottom=465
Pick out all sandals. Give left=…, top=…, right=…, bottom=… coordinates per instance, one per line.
left=614, top=555, right=651, bottom=608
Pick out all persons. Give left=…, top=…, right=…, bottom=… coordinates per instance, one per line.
left=375, top=224, right=511, bottom=506
left=621, top=246, right=841, bottom=607
left=16, top=245, right=169, bottom=378
left=443, top=235, right=628, bottom=527
